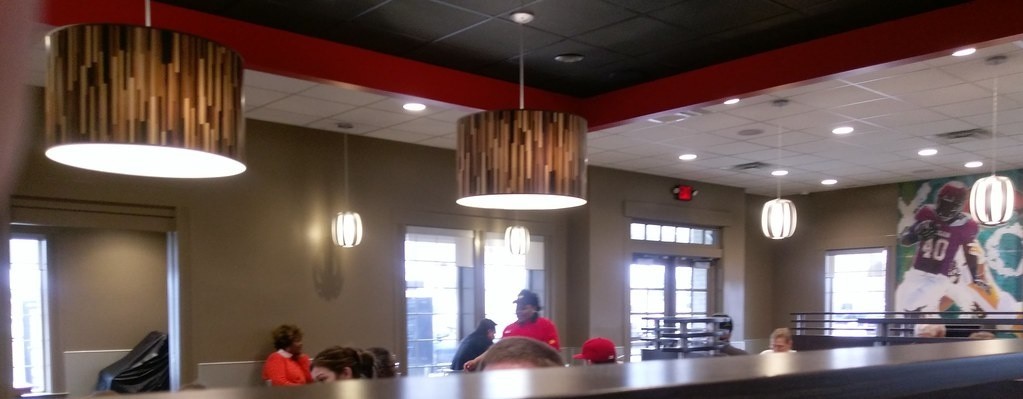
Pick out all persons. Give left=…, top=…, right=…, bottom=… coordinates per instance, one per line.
left=502, top=289, right=560, bottom=351
left=311, top=348, right=393, bottom=382
left=707, top=314, right=749, bottom=356
left=760, top=328, right=796, bottom=355
left=895, top=181, right=1018, bottom=338
left=261, top=325, right=313, bottom=387
left=463, top=337, right=564, bottom=372
left=572, top=337, right=617, bottom=365
left=450, top=318, right=497, bottom=370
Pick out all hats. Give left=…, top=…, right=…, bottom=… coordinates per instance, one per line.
left=573, top=338, right=615, bottom=361
left=513, top=289, right=538, bottom=305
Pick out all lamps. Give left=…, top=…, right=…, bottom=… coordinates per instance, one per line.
left=760, top=99, right=797, bottom=239
left=330, top=121, right=364, bottom=248
left=969, top=53, right=1015, bottom=226
left=673, top=185, right=699, bottom=201
left=456, top=12, right=587, bottom=210
left=505, top=208, right=533, bottom=257
left=43, top=0, right=249, bottom=178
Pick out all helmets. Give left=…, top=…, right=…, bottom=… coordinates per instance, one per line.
left=934, top=181, right=968, bottom=222
